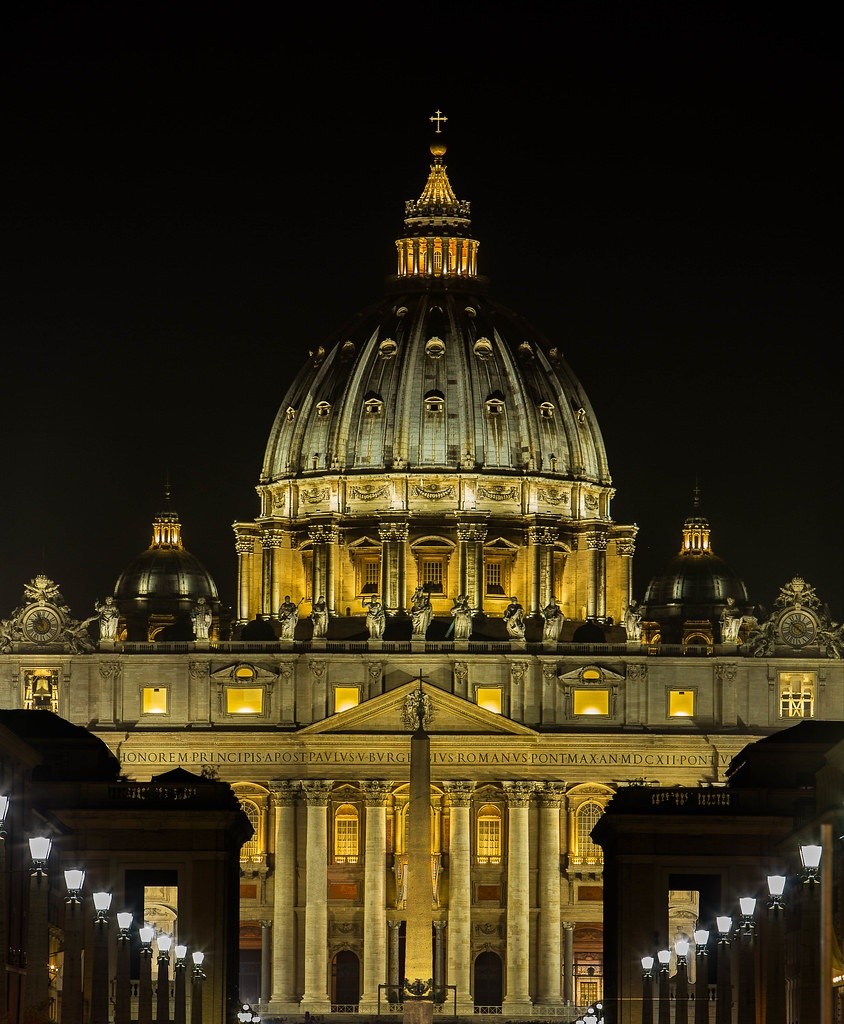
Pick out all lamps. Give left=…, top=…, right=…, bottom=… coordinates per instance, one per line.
left=641, top=838, right=823, bottom=980
left=679, top=692, right=684, bottom=695
left=153, top=689, right=159, bottom=692
left=0, top=790, right=207, bottom=985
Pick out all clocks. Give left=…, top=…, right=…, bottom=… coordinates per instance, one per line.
left=777, top=609, right=818, bottom=650
left=22, top=607, right=62, bottom=644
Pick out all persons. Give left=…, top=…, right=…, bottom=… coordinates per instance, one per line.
left=504, top=596, right=525, bottom=638
left=722, top=596, right=743, bottom=640
left=449, top=594, right=472, bottom=639
left=190, top=598, right=213, bottom=638
left=362, top=595, right=382, bottom=641
left=95, top=595, right=120, bottom=637
left=622, top=598, right=644, bottom=640
left=278, top=595, right=298, bottom=639
left=312, top=596, right=328, bottom=637
left=540, top=597, right=565, bottom=639
left=413, top=586, right=432, bottom=634
